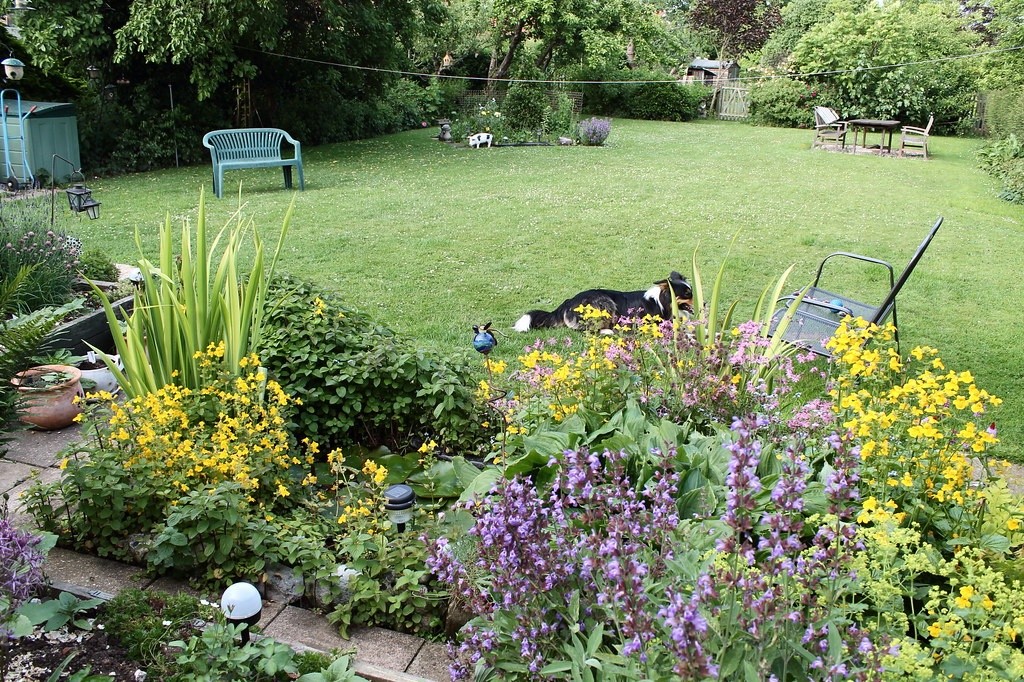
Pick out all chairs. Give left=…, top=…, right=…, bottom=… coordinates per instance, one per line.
left=898, top=116, right=934, bottom=158
left=768, top=216, right=944, bottom=387
left=814, top=107, right=848, bottom=148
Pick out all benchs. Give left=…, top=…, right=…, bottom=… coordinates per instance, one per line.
left=203, top=128, right=304, bottom=198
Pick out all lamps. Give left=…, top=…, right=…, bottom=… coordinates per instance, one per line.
left=221, top=582, right=263, bottom=646
left=383, top=484, right=416, bottom=534
left=0, top=43, right=26, bottom=81
left=50, top=154, right=102, bottom=244
left=537, top=130, right=542, bottom=141
left=87, top=64, right=100, bottom=78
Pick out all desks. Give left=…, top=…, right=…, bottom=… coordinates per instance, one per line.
left=849, top=119, right=901, bottom=155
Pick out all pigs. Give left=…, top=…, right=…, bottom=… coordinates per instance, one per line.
left=467, top=133, right=492, bottom=148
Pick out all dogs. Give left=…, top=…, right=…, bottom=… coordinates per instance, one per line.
left=514, top=271, right=695, bottom=332
